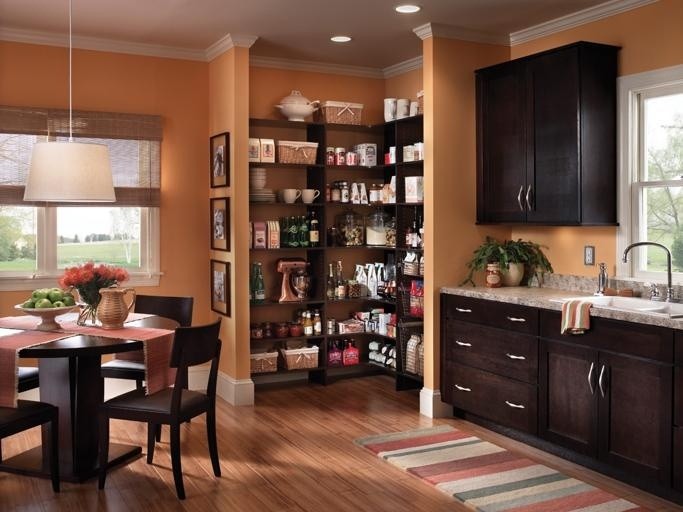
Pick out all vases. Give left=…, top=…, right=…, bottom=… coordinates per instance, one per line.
left=77, top=305, right=102, bottom=327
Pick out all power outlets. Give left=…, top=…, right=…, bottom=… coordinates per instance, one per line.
left=584, top=246, right=595, bottom=265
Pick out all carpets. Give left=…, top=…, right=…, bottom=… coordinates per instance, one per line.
left=355, top=423, right=653, bottom=512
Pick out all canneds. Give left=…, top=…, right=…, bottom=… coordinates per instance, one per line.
left=251, top=321, right=302, bottom=340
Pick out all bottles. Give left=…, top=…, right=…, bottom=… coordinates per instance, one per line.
left=326, top=316, right=336, bottom=336
left=406, top=205, right=423, bottom=249
left=325, top=146, right=346, bottom=165
left=296, top=308, right=322, bottom=336
left=249, top=261, right=265, bottom=305
left=280, top=211, right=319, bottom=247
left=484, top=262, right=502, bottom=288
left=326, top=260, right=345, bottom=300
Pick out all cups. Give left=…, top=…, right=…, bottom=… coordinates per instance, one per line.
left=282, top=188, right=300, bottom=203
left=300, top=188, right=320, bottom=203
left=396, top=99, right=411, bottom=120
left=383, top=98, right=397, bottom=123
left=410, top=101, right=419, bottom=117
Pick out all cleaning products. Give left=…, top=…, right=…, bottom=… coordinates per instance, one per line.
left=364, top=263, right=377, bottom=297
left=354, top=263, right=368, bottom=297
left=374, top=262, right=384, bottom=297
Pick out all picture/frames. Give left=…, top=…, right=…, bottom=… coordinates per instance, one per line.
left=210, top=132, right=230, bottom=188
left=210, top=258, right=230, bottom=317
left=210, top=197, right=230, bottom=252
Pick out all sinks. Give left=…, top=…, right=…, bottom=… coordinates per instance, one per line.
left=549, top=296, right=683, bottom=329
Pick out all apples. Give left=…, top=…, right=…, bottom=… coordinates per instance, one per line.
left=64, top=291, right=74, bottom=297
left=34, top=298, right=51, bottom=308
left=31, top=289, right=47, bottom=304
left=61, top=296, right=73, bottom=306
left=22, top=301, right=34, bottom=308
left=52, top=301, right=65, bottom=308
left=47, top=288, right=64, bottom=304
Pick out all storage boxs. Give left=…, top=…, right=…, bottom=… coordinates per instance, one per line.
left=250, top=347, right=278, bottom=373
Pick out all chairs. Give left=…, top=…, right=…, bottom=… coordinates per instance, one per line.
left=102, top=294, right=194, bottom=443
left=0, top=399, right=60, bottom=493
left=98, top=318, right=222, bottom=499
left=18, top=366, right=40, bottom=393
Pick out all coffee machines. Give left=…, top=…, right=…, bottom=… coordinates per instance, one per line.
left=275, top=257, right=311, bottom=303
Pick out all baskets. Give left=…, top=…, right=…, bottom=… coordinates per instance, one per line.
left=405, top=333, right=420, bottom=374
left=250, top=351, right=279, bottom=373
left=418, top=334, right=425, bottom=376
left=416, top=92, right=424, bottom=115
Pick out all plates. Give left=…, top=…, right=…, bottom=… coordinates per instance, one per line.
left=249, top=188, right=276, bottom=203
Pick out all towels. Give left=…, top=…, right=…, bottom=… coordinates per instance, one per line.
left=560, top=298, right=592, bottom=333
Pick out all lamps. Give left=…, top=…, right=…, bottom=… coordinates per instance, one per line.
left=22, top=0, right=116, bottom=202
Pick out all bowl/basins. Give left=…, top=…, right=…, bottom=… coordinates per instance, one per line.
left=249, top=167, right=267, bottom=190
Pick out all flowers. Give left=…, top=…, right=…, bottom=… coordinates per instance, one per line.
left=58, top=260, right=130, bottom=323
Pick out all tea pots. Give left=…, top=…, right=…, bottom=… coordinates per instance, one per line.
left=273, top=89, right=320, bottom=122
left=96, top=286, right=137, bottom=329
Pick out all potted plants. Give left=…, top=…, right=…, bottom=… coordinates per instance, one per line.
left=460, top=235, right=553, bottom=287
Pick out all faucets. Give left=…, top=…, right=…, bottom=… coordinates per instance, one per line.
left=622, top=241, right=680, bottom=302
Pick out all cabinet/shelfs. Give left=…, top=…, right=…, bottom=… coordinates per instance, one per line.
left=473, top=40, right=623, bottom=226
left=249, top=118, right=325, bottom=392
left=307, top=121, right=396, bottom=386
left=396, top=114, right=424, bottom=392
left=539, top=308, right=674, bottom=489
left=439, top=293, right=540, bottom=436
